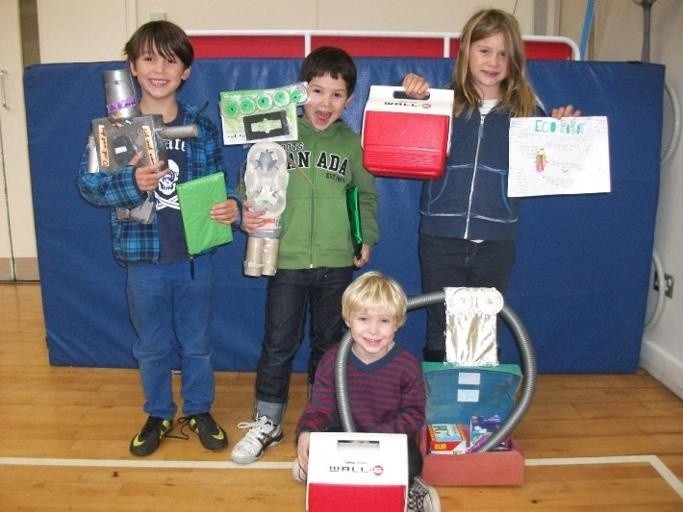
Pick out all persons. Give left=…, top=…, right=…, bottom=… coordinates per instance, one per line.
left=77, top=20, right=243, bottom=456
left=399, top=9, right=581, bottom=361
left=293, top=271, right=441, bottom=511
left=232, top=44, right=380, bottom=462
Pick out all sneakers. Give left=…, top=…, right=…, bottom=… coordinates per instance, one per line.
left=187, top=412, right=227, bottom=450
left=407, top=476, right=441, bottom=512
left=291, top=456, right=306, bottom=485
left=129, top=416, right=174, bottom=457
left=229, top=416, right=283, bottom=464
